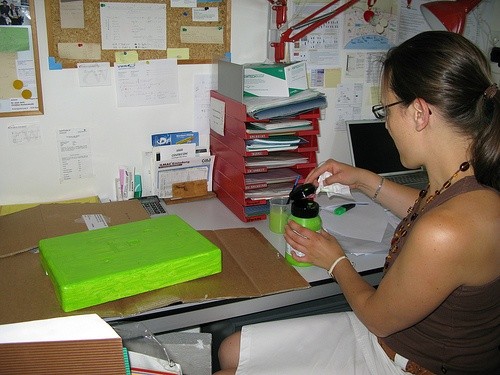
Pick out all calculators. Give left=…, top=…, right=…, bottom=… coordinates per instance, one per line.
left=128, top=195, right=168, bottom=218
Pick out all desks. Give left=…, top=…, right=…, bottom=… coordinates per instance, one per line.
left=0, top=193, right=386, bottom=375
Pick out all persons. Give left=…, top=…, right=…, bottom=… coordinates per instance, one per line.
left=0, top=0, right=23, bottom=25
left=212, top=31, right=500, bottom=375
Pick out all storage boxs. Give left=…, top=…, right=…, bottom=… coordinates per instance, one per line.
left=238, top=61, right=308, bottom=98
left=37, top=215, right=222, bottom=312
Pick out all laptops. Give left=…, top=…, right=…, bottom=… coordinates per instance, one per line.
left=346, top=119, right=429, bottom=189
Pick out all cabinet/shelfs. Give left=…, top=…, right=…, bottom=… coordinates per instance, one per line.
left=209, top=90, right=321, bottom=225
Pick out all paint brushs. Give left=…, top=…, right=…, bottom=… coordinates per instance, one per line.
left=284, top=175, right=300, bottom=212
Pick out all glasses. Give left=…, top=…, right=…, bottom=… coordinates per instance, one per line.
left=372, top=99, right=432, bottom=120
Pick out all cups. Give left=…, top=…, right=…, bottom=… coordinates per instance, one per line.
left=270, top=198, right=291, bottom=234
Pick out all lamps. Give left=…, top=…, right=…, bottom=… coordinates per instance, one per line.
left=273, top=0, right=485, bottom=64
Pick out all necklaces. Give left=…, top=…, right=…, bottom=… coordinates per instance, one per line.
left=384, top=160, right=473, bottom=273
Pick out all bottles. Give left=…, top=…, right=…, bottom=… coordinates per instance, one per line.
left=285, top=182, right=323, bottom=267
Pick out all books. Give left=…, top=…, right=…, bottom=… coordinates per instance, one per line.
left=245, top=120, right=313, bottom=201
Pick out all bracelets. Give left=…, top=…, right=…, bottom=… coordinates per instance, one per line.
left=328, top=255, right=348, bottom=279
left=372, top=177, right=385, bottom=200
